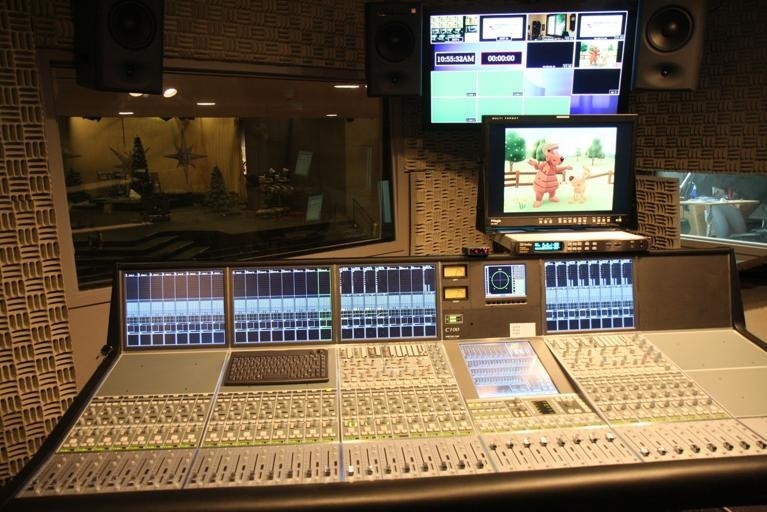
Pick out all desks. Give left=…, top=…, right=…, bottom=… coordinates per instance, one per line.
left=679, top=197, right=760, bottom=237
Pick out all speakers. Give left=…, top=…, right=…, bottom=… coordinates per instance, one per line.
left=74, top=0, right=166, bottom=94
left=633, top=1, right=707, bottom=93
left=364, top=1, right=421, bottom=97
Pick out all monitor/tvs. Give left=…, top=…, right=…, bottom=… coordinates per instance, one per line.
left=481, top=115, right=635, bottom=230
left=417, top=0, right=636, bottom=130
